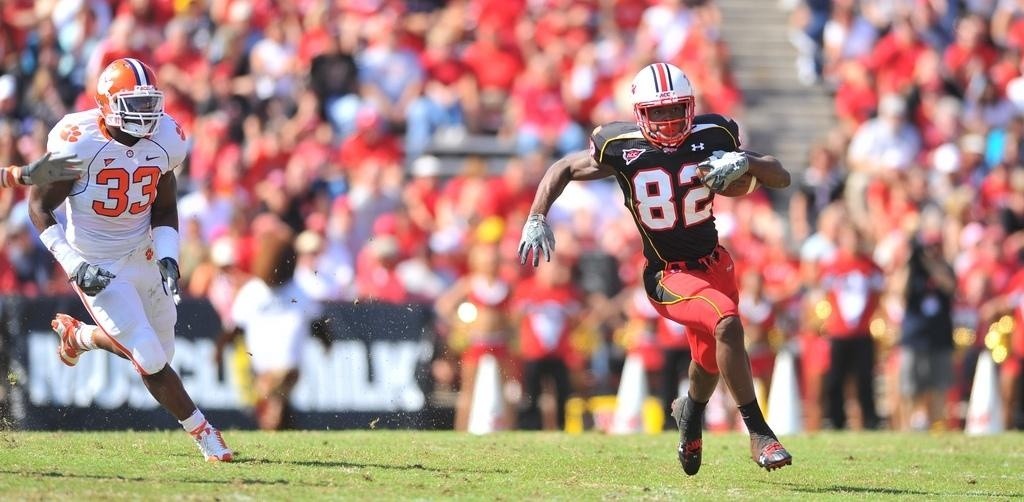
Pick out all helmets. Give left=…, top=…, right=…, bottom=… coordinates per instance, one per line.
left=630, top=62, right=696, bottom=152
left=94, top=59, right=166, bottom=139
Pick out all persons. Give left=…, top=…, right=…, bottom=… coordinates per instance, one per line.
left=28, top=59, right=236, bottom=462
left=1, top=0, right=1024, bottom=429
left=517, top=61, right=791, bottom=475
left=0, top=151, right=84, bottom=188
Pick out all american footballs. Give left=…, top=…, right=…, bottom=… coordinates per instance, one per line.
left=696, top=166, right=762, bottom=196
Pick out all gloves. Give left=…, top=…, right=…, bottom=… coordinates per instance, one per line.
left=24, top=151, right=84, bottom=187
left=517, top=214, right=556, bottom=266
left=154, top=257, right=183, bottom=307
left=68, top=263, right=116, bottom=298
left=698, top=152, right=749, bottom=192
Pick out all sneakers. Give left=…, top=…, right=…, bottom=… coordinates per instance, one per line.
left=672, top=395, right=709, bottom=477
left=51, top=312, right=87, bottom=366
left=749, top=428, right=792, bottom=469
left=190, top=418, right=233, bottom=464
